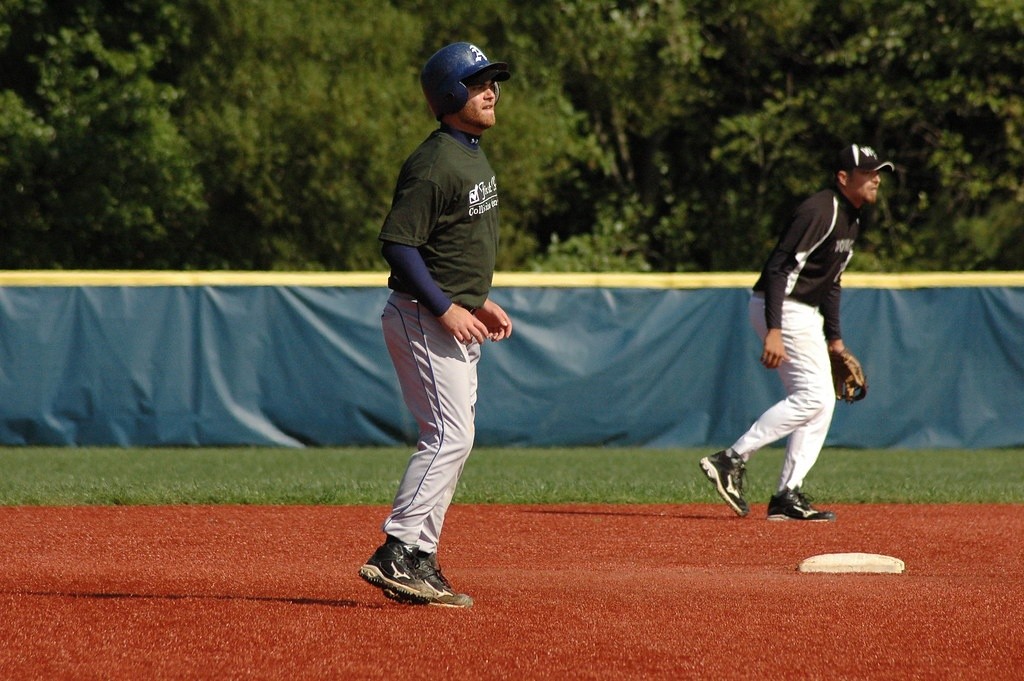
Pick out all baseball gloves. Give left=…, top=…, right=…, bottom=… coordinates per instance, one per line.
left=830, top=348, right=869, bottom=404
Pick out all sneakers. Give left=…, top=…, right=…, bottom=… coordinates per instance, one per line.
left=357, top=534, right=434, bottom=605
left=381, top=552, right=473, bottom=608
left=700, top=447, right=749, bottom=517
left=767, top=485, right=834, bottom=522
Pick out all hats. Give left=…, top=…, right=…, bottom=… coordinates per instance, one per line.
left=834, top=144, right=894, bottom=179
left=463, top=67, right=511, bottom=86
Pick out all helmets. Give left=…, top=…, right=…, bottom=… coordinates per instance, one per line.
left=420, top=42, right=509, bottom=121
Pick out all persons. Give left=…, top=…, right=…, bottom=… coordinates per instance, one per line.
left=359, top=41, right=515, bottom=609
left=701, top=143, right=894, bottom=523
